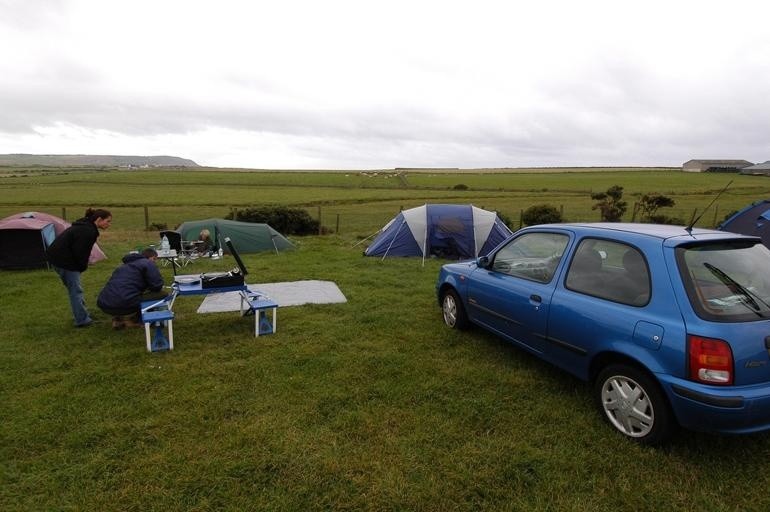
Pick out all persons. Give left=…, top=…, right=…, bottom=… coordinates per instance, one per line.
left=46, top=209, right=165, bottom=330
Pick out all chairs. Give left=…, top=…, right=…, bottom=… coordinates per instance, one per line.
left=568, top=245, right=648, bottom=304
left=159, top=230, right=194, bottom=269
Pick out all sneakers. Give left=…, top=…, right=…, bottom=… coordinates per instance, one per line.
left=112, top=319, right=144, bottom=329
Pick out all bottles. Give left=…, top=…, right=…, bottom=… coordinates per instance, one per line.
left=162, top=236, right=170, bottom=255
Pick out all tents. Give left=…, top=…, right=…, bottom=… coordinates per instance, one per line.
left=363, top=202, right=513, bottom=262
left=717, top=198, right=770, bottom=249
left=175, top=219, right=297, bottom=256
left=0, top=212, right=109, bottom=269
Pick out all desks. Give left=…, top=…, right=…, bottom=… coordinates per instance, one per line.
left=169, top=273, right=255, bottom=310
left=155, top=248, right=177, bottom=275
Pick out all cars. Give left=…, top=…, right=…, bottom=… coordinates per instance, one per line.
left=437, top=181, right=770, bottom=447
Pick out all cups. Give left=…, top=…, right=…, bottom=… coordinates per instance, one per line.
left=149, top=245, right=156, bottom=251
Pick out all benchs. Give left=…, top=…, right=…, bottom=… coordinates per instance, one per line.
left=140, top=294, right=174, bottom=354
left=239, top=288, right=278, bottom=337
